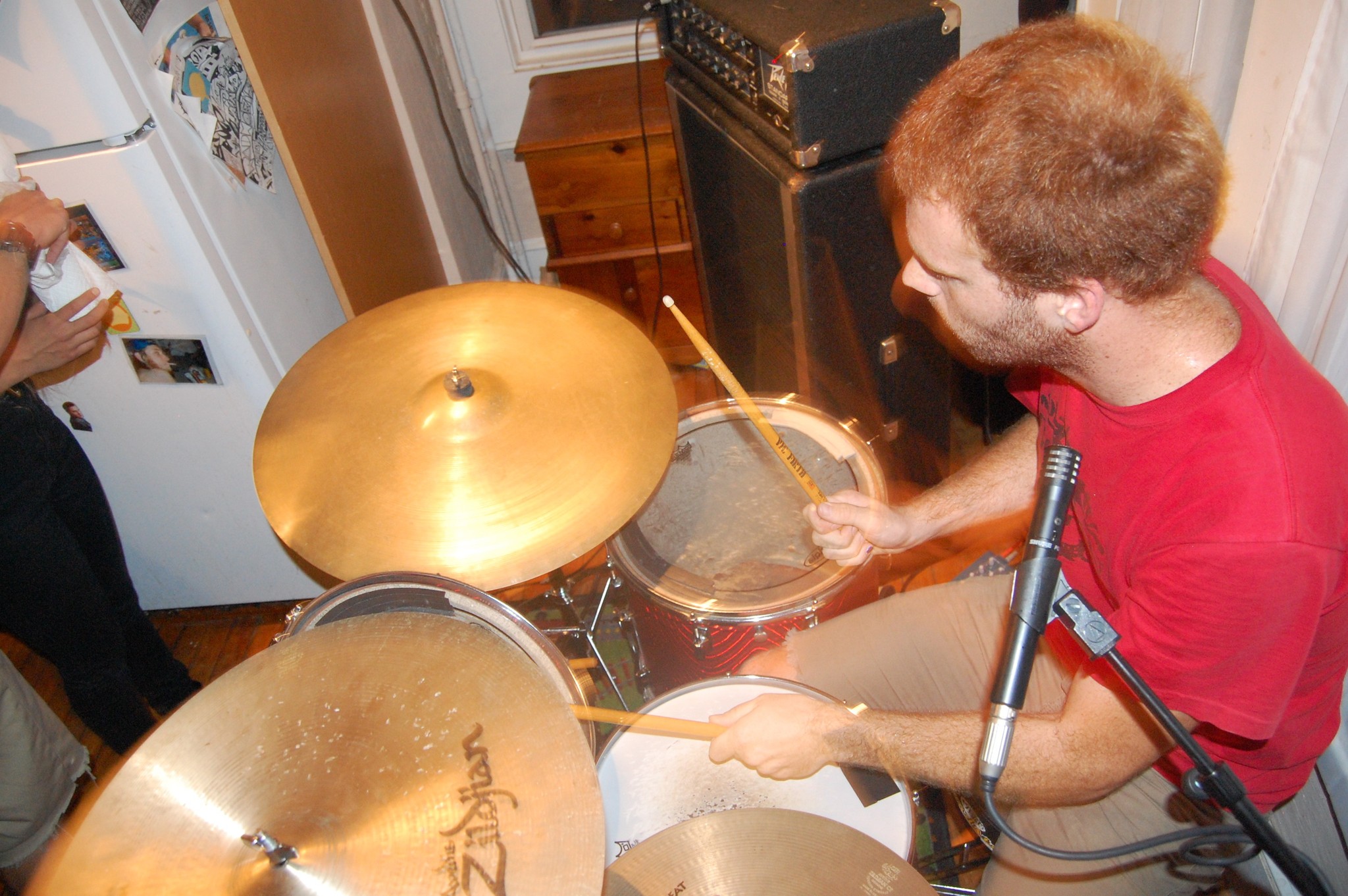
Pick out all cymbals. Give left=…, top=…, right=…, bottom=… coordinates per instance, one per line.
left=600, top=807, right=941, bottom=896
left=248, top=280, right=681, bottom=594
left=24, top=610, right=606, bottom=896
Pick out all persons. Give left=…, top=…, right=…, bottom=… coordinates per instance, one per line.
left=708, top=15, right=1348, bottom=896
left=127, top=340, right=175, bottom=384
left=61, top=401, right=91, bottom=431
left=0, top=190, right=206, bottom=755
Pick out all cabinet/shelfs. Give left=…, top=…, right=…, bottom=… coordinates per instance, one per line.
left=513, top=57, right=717, bottom=410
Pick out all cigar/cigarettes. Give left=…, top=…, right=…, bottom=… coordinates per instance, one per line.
left=168, top=363, right=177, bottom=365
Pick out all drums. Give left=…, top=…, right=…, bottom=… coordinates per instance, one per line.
left=593, top=673, right=919, bottom=869
left=598, top=398, right=900, bottom=698
left=278, top=568, right=598, bottom=762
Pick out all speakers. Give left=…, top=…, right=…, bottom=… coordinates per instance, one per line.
left=659, top=74, right=952, bottom=486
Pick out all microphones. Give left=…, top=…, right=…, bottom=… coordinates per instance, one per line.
left=976, top=445, right=1080, bottom=789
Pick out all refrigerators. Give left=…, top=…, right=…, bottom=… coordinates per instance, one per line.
left=0, top=0, right=348, bottom=612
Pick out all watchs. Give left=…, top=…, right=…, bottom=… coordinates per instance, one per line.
left=0, top=219, right=40, bottom=268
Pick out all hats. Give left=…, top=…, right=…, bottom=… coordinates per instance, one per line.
left=131, top=338, right=157, bottom=352
